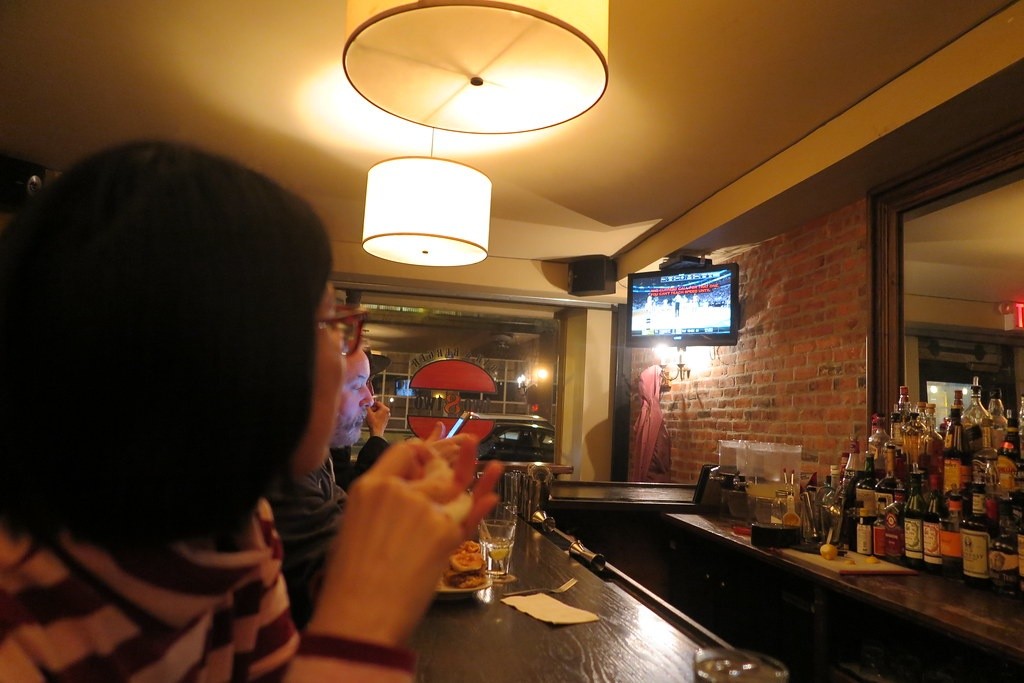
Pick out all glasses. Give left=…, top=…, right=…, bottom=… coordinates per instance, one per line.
left=317, top=304, right=368, bottom=359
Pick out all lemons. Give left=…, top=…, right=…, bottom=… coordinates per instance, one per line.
left=820, top=544, right=838, bottom=559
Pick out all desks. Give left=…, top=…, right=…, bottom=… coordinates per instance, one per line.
left=475, top=460, right=574, bottom=479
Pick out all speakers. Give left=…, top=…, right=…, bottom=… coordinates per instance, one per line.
left=568, top=259, right=616, bottom=297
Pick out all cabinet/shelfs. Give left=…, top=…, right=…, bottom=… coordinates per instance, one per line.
left=665, top=512, right=1024, bottom=683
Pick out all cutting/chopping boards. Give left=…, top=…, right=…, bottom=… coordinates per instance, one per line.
left=775, top=549, right=917, bottom=575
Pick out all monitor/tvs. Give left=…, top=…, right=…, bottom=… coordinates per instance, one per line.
left=626, top=262, right=739, bottom=348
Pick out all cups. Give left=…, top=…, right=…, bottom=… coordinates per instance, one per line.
left=693, top=647, right=790, bottom=682
left=479, top=473, right=519, bottom=576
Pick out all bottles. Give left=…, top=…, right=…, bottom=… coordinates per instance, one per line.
left=832, top=383, right=1024, bottom=596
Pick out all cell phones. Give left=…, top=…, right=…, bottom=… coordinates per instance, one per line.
left=445, top=411, right=471, bottom=441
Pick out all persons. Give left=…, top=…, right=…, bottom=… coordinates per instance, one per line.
left=0, top=140, right=499, bottom=683
left=269, top=342, right=468, bottom=629
left=632, top=283, right=731, bottom=323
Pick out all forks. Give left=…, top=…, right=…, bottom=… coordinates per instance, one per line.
left=503, top=578, right=576, bottom=597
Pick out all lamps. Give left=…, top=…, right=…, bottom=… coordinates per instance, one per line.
left=342, top=0, right=611, bottom=136
left=361, top=129, right=492, bottom=267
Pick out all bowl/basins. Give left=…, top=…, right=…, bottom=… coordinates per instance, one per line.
left=436, top=578, right=492, bottom=600
